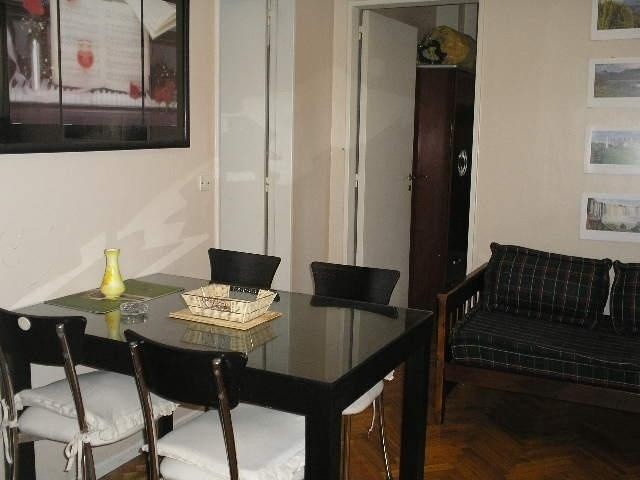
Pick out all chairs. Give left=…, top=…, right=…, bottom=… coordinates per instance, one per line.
left=1, top=300, right=181, bottom=480
left=124, top=328, right=304, bottom=480
left=310, top=261, right=399, bottom=479
left=208, top=247, right=280, bottom=290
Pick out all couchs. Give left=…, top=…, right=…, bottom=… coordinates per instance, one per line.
left=425, top=241, right=640, bottom=428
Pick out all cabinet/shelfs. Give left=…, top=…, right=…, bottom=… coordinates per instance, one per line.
left=407, top=61, right=476, bottom=329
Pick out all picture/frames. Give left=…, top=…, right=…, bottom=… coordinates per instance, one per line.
left=578, top=1, right=640, bottom=243
left=0, top=0, right=190, bottom=155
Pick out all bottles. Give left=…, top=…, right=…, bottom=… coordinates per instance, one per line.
left=105, top=310, right=123, bottom=342
left=99, top=248, right=125, bottom=299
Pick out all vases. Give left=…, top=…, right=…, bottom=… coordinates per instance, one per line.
left=100, top=249, right=126, bottom=298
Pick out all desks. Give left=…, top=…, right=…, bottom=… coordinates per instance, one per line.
left=1, top=271, right=436, bottom=479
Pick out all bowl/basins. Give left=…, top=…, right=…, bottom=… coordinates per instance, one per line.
left=181, top=283, right=278, bottom=323
left=179, top=321, right=278, bottom=356
